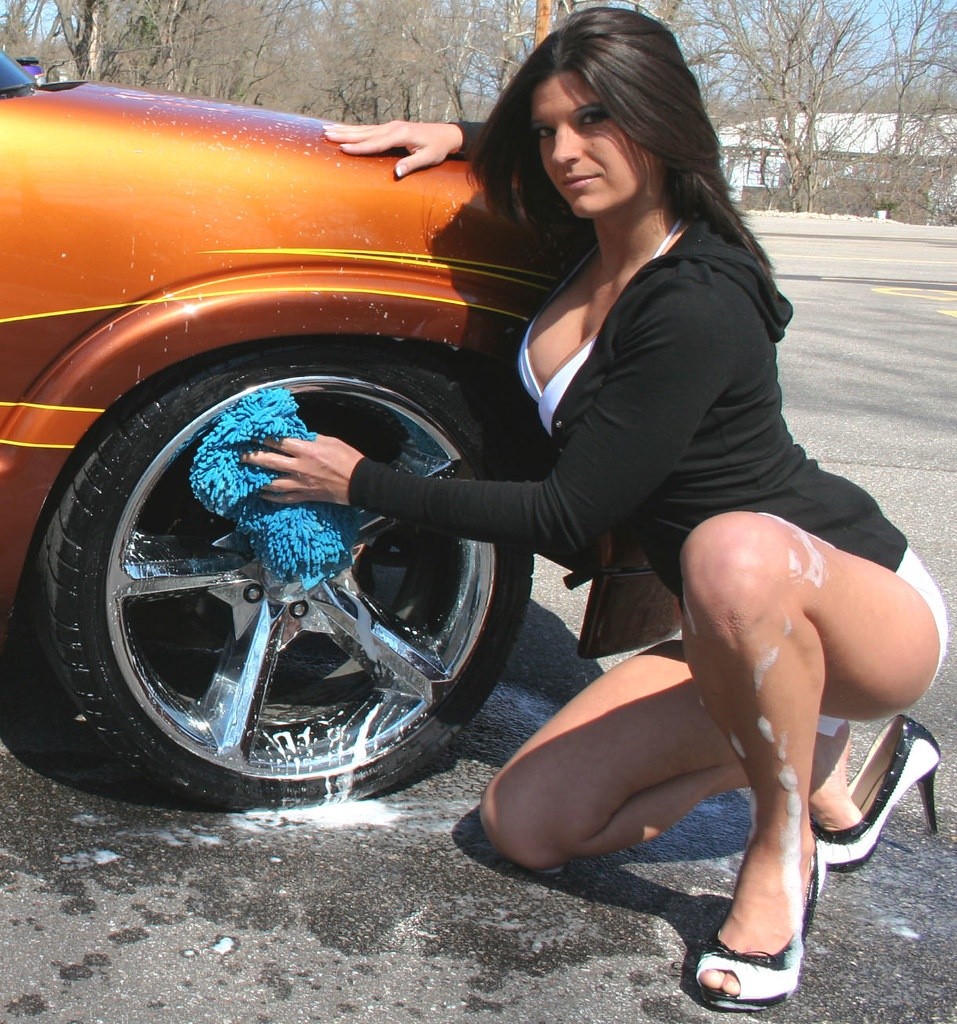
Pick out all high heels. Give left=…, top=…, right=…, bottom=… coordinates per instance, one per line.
left=697, top=832, right=823, bottom=1013
left=808, top=714, right=942, bottom=872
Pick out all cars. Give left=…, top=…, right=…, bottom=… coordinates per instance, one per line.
left=0, top=45, right=599, bottom=813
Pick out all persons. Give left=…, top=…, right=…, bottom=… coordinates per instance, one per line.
left=239, top=5, right=949, bottom=1011
